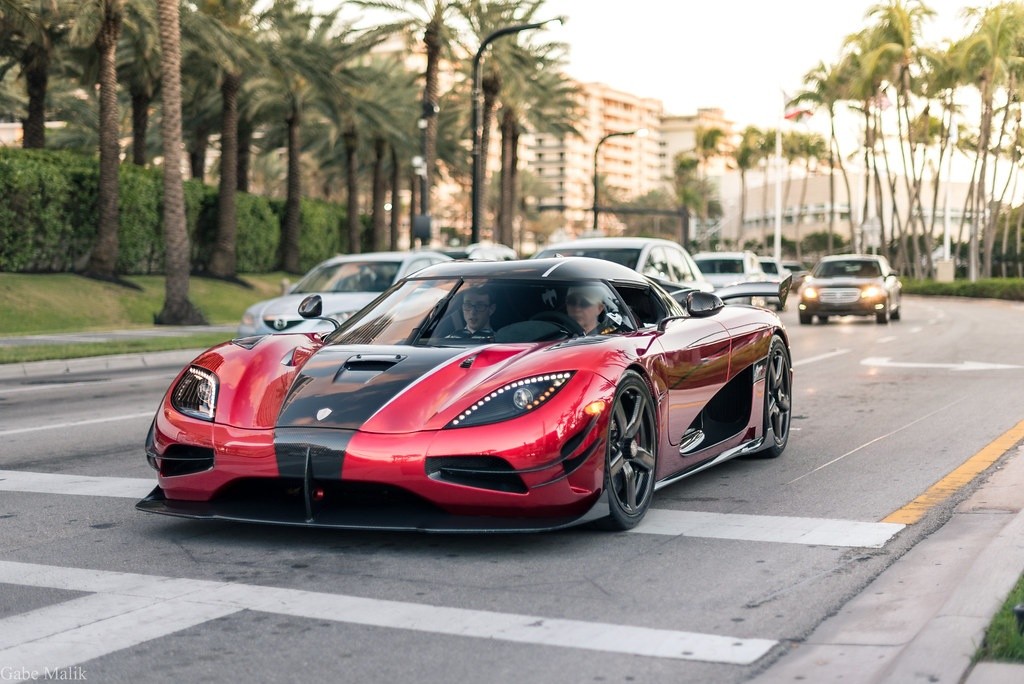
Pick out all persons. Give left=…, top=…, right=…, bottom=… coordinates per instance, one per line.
left=445, top=289, right=495, bottom=338
left=566, top=286, right=616, bottom=336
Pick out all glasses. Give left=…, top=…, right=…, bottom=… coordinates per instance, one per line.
left=461, top=303, right=490, bottom=312
left=566, top=297, right=591, bottom=308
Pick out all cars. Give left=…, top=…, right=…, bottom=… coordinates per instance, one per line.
left=529, top=237, right=718, bottom=305
left=417, top=241, right=805, bottom=315
left=236, top=251, right=470, bottom=347
left=799, top=254, right=902, bottom=326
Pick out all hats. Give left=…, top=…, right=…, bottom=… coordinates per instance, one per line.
left=567, top=283, right=605, bottom=304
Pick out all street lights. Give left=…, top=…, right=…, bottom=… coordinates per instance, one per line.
left=591, top=125, right=654, bottom=238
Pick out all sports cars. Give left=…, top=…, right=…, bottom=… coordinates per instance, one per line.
left=135, top=253, right=794, bottom=535
